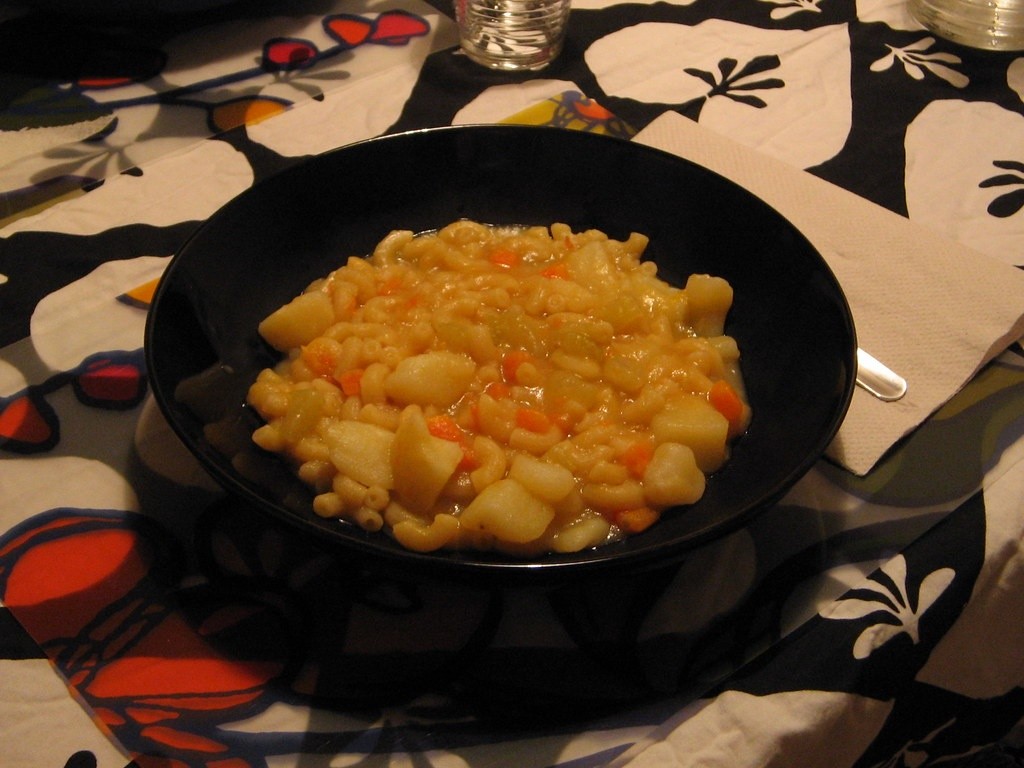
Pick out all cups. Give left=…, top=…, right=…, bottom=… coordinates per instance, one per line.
left=454, top=0, right=571, bottom=71
left=908, top=0, right=1024, bottom=51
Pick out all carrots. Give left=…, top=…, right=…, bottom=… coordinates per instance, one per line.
left=299, top=248, right=742, bottom=531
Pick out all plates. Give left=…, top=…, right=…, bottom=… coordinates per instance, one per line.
left=147, top=124, right=858, bottom=570
